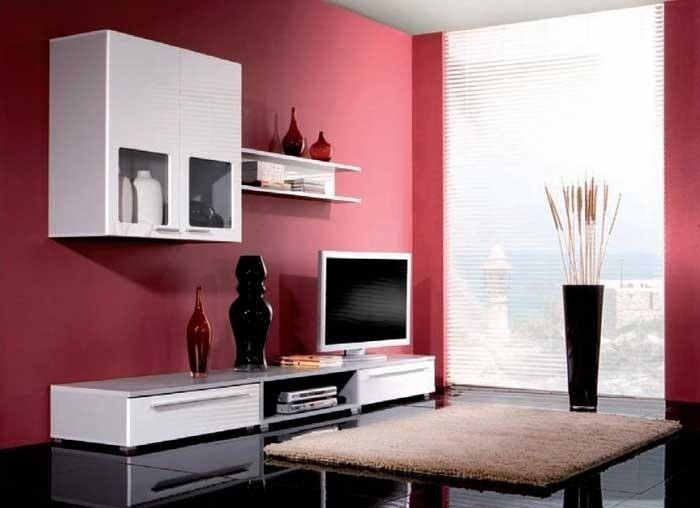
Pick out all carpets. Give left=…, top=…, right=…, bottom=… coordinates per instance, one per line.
left=265, top=400, right=684, bottom=489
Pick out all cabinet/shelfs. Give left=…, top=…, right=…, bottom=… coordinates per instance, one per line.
left=48, top=28, right=243, bottom=244
left=50, top=354, right=436, bottom=455
left=51, top=401, right=440, bottom=508
left=241, top=147, right=363, bottom=204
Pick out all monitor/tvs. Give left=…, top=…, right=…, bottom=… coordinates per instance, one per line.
left=317, top=249, right=412, bottom=362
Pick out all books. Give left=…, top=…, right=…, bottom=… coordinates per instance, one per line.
left=269, top=355, right=344, bottom=368
left=284, top=178, right=326, bottom=195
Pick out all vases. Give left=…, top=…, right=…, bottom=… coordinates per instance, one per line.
left=564, top=473, right=603, bottom=508
left=118, top=174, right=133, bottom=222
left=563, top=284, right=602, bottom=410
left=186, top=286, right=212, bottom=378
left=229, top=255, right=273, bottom=372
left=283, top=107, right=305, bottom=156
left=310, top=131, right=333, bottom=162
left=134, top=171, right=163, bottom=226
